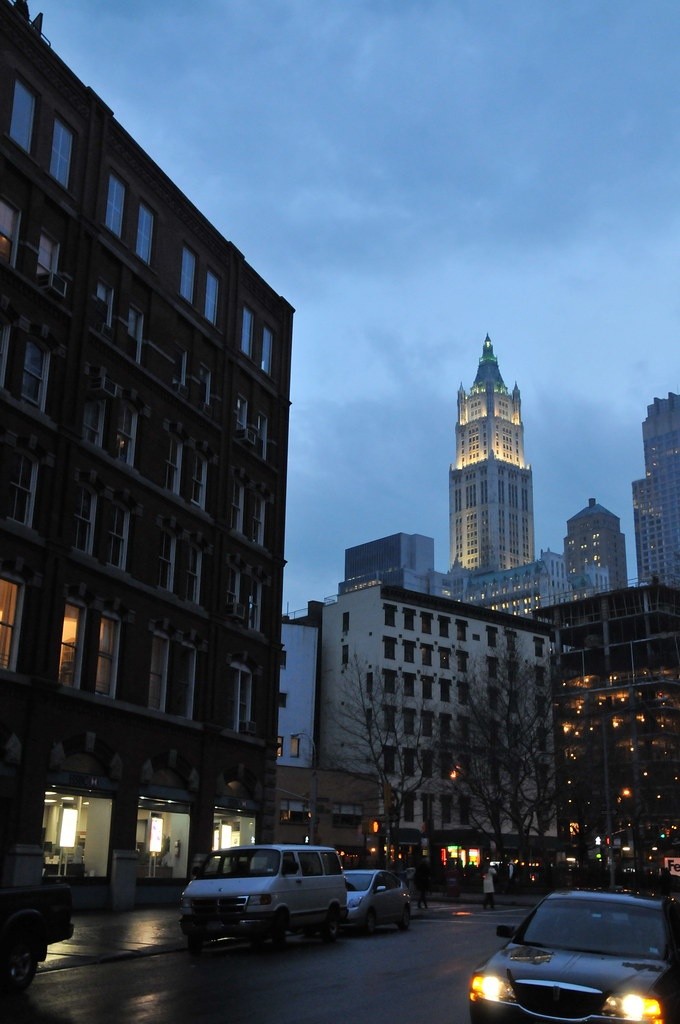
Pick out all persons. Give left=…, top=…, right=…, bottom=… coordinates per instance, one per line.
left=414, top=859, right=673, bottom=909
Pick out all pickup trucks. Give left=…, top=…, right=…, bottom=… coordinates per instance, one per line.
left=0, top=878, right=76, bottom=994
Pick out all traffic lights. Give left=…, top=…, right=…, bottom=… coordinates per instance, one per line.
left=659, top=831, right=666, bottom=839
left=594, top=833, right=603, bottom=847
left=368, top=818, right=380, bottom=834
left=604, top=832, right=613, bottom=847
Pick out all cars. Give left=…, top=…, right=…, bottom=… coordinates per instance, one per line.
left=467, top=886, right=680, bottom=1024
left=308, top=868, right=412, bottom=934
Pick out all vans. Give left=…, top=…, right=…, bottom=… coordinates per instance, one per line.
left=176, top=843, right=350, bottom=957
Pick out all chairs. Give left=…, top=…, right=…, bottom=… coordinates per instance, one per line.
left=600, top=924, right=636, bottom=949
left=274, top=858, right=291, bottom=873
left=232, top=861, right=250, bottom=875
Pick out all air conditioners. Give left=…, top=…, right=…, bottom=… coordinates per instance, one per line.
left=95, top=322, right=115, bottom=342
left=240, top=721, right=256, bottom=735
left=88, top=376, right=118, bottom=400
left=39, top=272, right=67, bottom=300
left=226, top=603, right=245, bottom=620
left=236, top=429, right=256, bottom=447
left=171, top=383, right=189, bottom=398
left=199, top=402, right=212, bottom=416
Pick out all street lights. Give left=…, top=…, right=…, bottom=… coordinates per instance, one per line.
left=599, top=695, right=668, bottom=892
left=289, top=731, right=316, bottom=846
